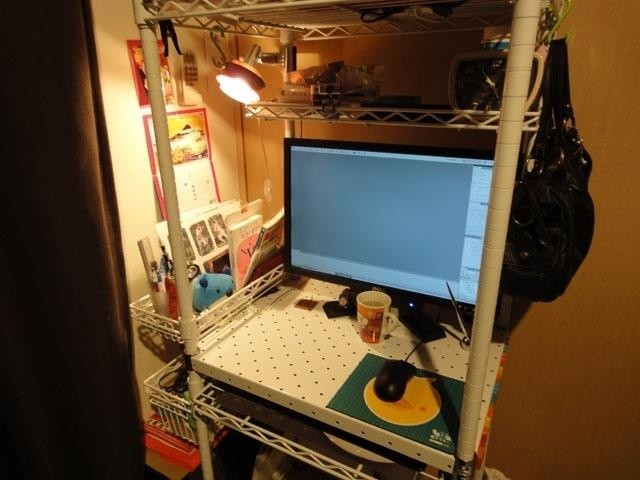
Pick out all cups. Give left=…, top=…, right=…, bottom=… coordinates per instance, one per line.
left=357, top=292, right=396, bottom=344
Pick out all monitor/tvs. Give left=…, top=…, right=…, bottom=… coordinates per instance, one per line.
left=281, top=135, right=494, bottom=345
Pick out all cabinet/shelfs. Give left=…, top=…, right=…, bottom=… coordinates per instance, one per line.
left=130, top=1, right=542, bottom=480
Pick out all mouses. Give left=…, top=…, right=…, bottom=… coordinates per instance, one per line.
left=374, top=358, right=417, bottom=401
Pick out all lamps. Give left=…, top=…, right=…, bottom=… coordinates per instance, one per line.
left=214, top=43, right=284, bottom=105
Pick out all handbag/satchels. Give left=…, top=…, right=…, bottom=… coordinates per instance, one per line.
left=502, top=29, right=596, bottom=303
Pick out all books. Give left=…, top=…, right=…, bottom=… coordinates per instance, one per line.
left=227, top=209, right=287, bottom=292
